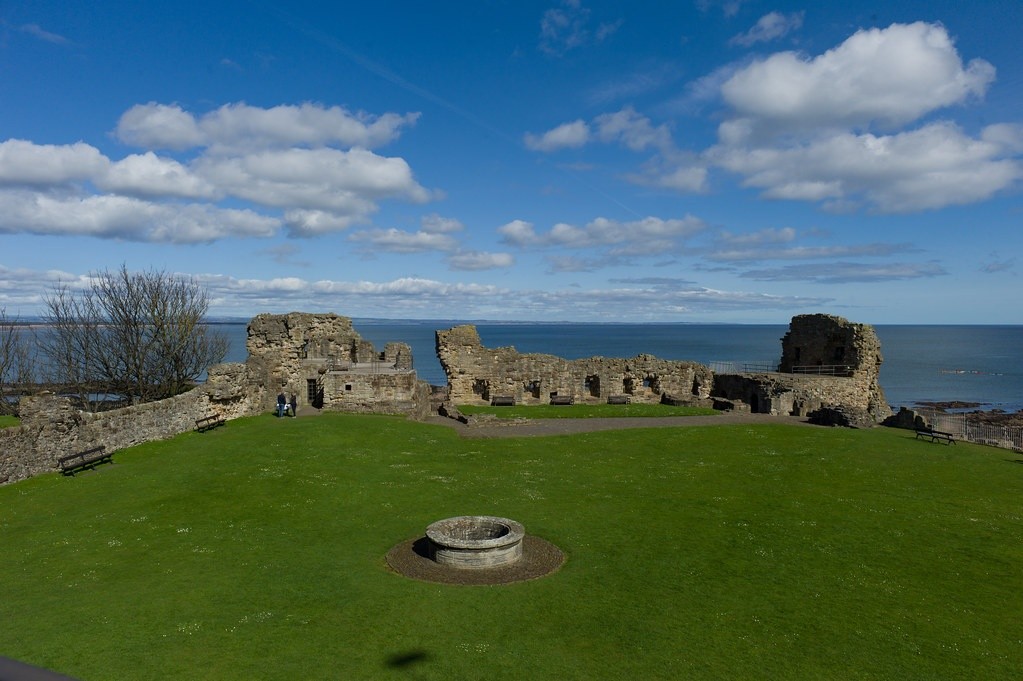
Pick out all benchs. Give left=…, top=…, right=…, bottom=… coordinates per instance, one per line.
left=914, top=427, right=956, bottom=446
left=195, top=413, right=227, bottom=434
left=57, top=446, right=114, bottom=477
left=274, top=403, right=291, bottom=415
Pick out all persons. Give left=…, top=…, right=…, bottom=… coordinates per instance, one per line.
left=278, top=391, right=286, bottom=418
left=287, top=392, right=297, bottom=418
